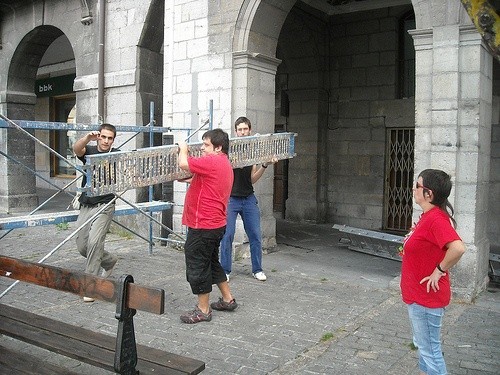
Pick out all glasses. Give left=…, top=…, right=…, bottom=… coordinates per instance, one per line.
left=416, top=182, right=429, bottom=190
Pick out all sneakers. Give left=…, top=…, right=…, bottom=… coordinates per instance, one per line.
left=211, top=297, right=237, bottom=310
left=180, top=306, right=212, bottom=324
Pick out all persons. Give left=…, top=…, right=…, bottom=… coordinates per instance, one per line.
left=219, top=116, right=271, bottom=283
left=400, top=169, right=466, bottom=375
left=176, top=130, right=238, bottom=323
left=74, top=123, right=121, bottom=302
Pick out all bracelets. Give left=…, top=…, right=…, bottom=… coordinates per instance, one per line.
left=262, top=165, right=267, bottom=169
left=436, top=264, right=446, bottom=273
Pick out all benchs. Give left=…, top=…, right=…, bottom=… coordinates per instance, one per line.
left=0, top=255, right=205, bottom=375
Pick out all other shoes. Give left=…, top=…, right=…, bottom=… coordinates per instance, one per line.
left=101, top=267, right=113, bottom=278
left=83, top=296, right=94, bottom=302
left=252, top=272, right=266, bottom=280
left=226, top=274, right=230, bottom=282
